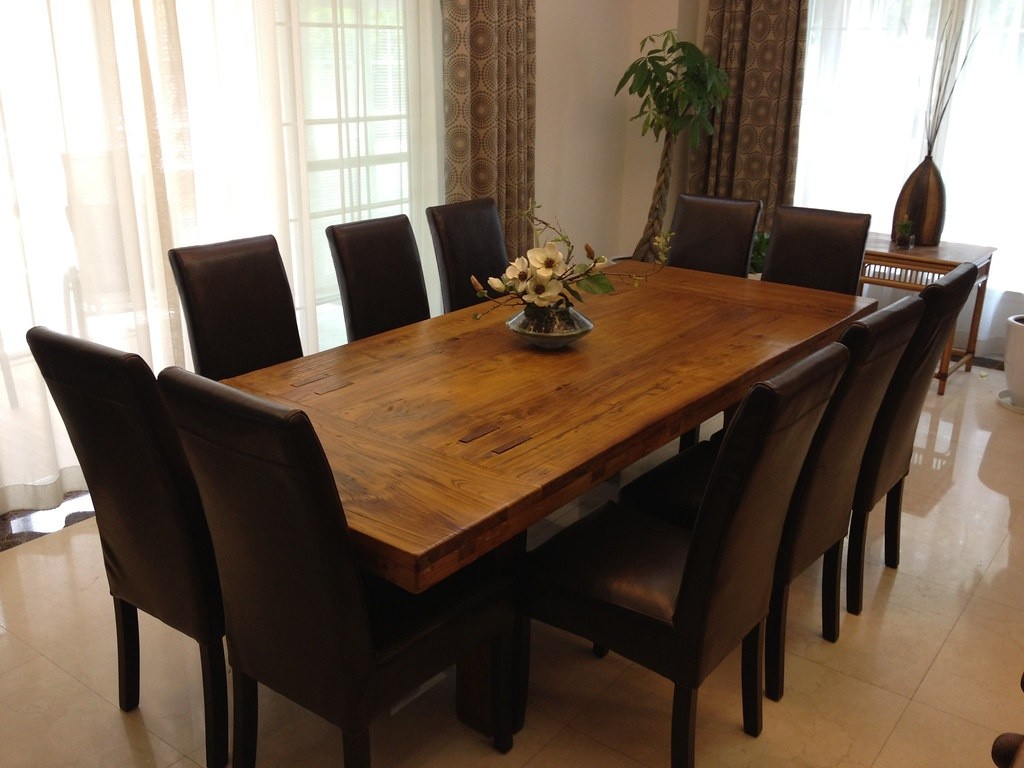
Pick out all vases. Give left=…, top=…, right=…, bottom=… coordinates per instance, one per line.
left=505, top=305, right=595, bottom=349
left=889, top=155, right=948, bottom=247
left=994, top=315, right=1024, bottom=415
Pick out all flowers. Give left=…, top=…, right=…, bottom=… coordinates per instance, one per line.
left=458, top=198, right=676, bottom=320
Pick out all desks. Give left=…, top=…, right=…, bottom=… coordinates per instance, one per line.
left=854, top=231, right=997, bottom=396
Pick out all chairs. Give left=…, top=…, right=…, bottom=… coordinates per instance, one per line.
left=168, top=234, right=305, bottom=381
left=27, top=326, right=231, bottom=768
left=323, top=213, right=431, bottom=345
left=619, top=291, right=926, bottom=699
left=157, top=365, right=516, bottom=768
left=512, top=340, right=849, bottom=768
left=661, top=194, right=763, bottom=279
left=707, top=260, right=980, bottom=618
left=759, top=206, right=873, bottom=296
left=424, top=195, right=513, bottom=316
